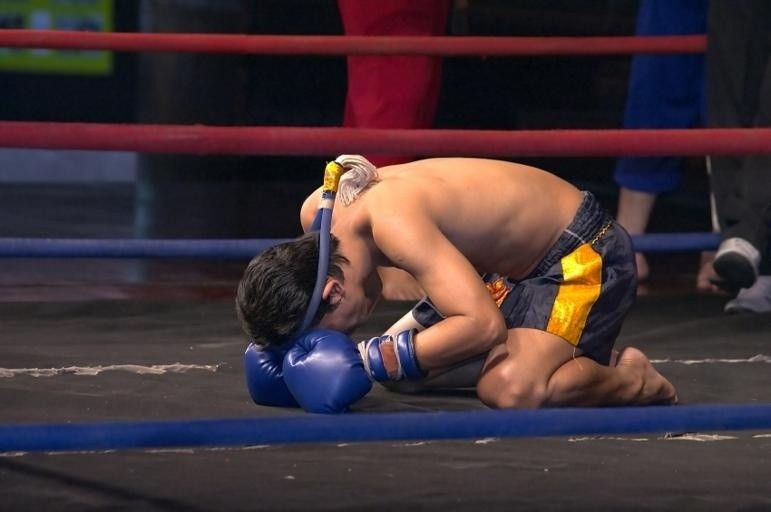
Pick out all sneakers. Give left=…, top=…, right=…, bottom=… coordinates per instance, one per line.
left=721, top=274, right=771, bottom=315
left=712, top=236, right=762, bottom=294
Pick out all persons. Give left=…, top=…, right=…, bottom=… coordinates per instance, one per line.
left=233, top=153, right=681, bottom=411
left=610, top=0, right=730, bottom=297
left=699, top=0, right=771, bottom=318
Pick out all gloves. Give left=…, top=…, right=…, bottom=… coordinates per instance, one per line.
left=243, top=339, right=300, bottom=405
left=282, top=329, right=426, bottom=415
